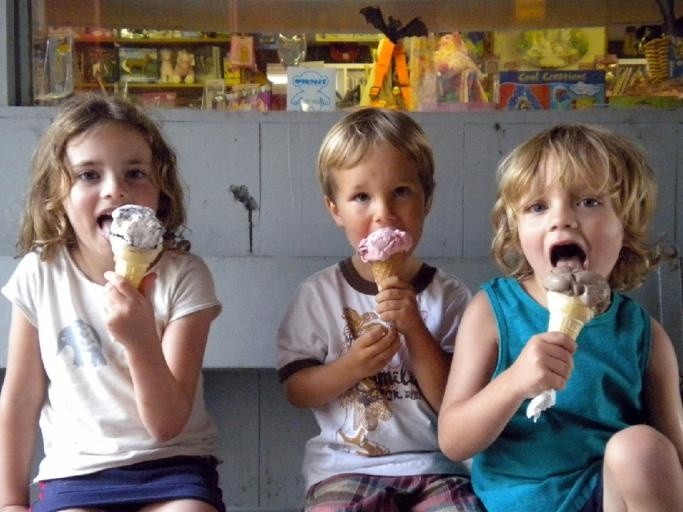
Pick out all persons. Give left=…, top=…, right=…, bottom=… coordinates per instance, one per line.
left=274, top=107, right=490, bottom=512
left=0, top=93, right=223, bottom=511
left=437, top=121, right=682, bottom=511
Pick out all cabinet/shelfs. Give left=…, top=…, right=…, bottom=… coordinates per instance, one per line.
left=70, top=37, right=259, bottom=88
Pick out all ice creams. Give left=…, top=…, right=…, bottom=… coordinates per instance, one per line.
left=109, top=204, right=165, bottom=291
left=357, top=226, right=413, bottom=291
left=543, top=264, right=612, bottom=340
